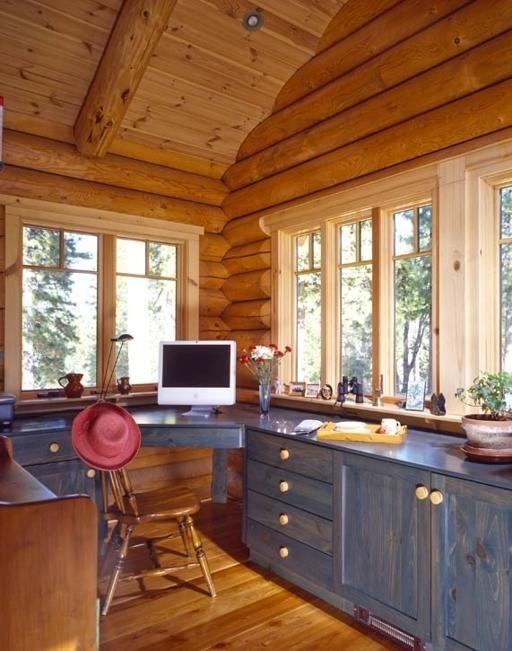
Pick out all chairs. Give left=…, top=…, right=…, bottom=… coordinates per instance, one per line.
left=99, top=464, right=217, bottom=614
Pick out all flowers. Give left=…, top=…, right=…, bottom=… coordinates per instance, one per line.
left=454, top=369, right=512, bottom=421
left=236, top=343, right=292, bottom=382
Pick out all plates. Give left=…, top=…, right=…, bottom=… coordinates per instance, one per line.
left=331, top=427, right=371, bottom=435
left=458, top=441, right=511, bottom=465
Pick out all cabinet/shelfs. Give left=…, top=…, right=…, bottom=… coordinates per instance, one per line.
left=0, top=436, right=97, bottom=651
left=334, top=449, right=512, bottom=649
left=245, top=426, right=334, bottom=586
left=0, top=411, right=103, bottom=540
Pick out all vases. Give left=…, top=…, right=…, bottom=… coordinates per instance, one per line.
left=258, top=384, right=270, bottom=415
left=457, top=414, right=512, bottom=461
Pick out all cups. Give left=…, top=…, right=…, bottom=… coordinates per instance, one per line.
left=381, top=419, right=401, bottom=436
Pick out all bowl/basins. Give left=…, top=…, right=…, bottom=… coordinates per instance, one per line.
left=335, top=422, right=368, bottom=432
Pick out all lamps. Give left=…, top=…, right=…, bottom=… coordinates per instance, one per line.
left=100, top=333, right=134, bottom=396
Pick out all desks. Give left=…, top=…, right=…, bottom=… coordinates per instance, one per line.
left=1, top=403, right=512, bottom=646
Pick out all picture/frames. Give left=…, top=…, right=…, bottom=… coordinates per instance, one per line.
left=306, top=385, right=320, bottom=395
left=289, top=381, right=305, bottom=396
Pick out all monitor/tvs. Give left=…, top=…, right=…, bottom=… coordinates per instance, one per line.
left=158, top=340, right=236, bottom=416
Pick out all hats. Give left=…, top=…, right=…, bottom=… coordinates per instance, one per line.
left=71, top=402, right=142, bottom=472
